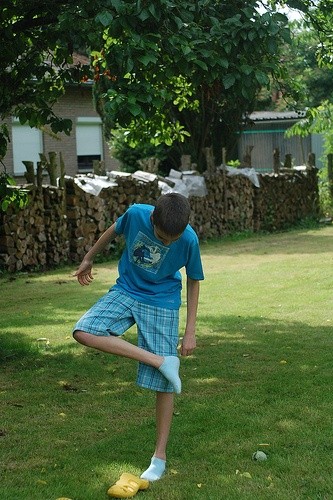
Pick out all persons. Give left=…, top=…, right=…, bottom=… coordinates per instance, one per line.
left=71, top=193, right=204, bottom=482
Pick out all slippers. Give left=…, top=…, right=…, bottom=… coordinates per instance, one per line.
left=107, top=472, right=149, bottom=498
